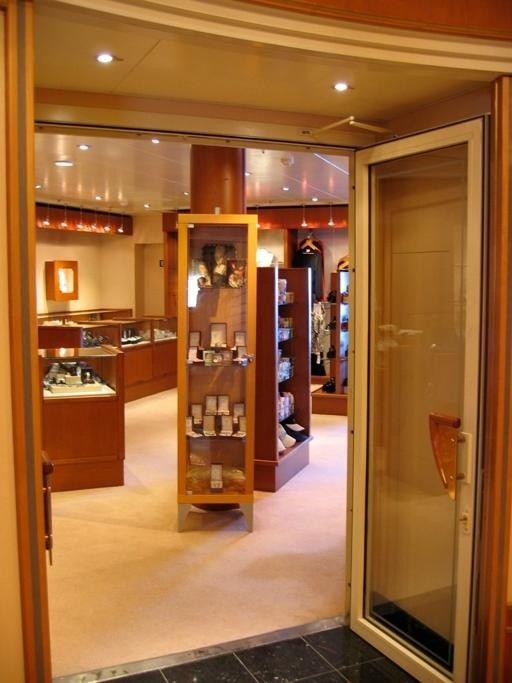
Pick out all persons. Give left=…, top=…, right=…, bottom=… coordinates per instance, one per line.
left=300, top=230, right=322, bottom=299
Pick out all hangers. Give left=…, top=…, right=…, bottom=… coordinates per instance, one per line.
left=295, top=228, right=324, bottom=257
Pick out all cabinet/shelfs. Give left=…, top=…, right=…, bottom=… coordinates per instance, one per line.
left=256, top=267, right=315, bottom=492
left=177, top=214, right=255, bottom=532
left=35, top=307, right=181, bottom=403
left=310, top=264, right=352, bottom=416
left=36, top=345, right=127, bottom=491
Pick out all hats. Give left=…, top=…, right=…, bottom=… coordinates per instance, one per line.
left=276, top=416, right=307, bottom=456
